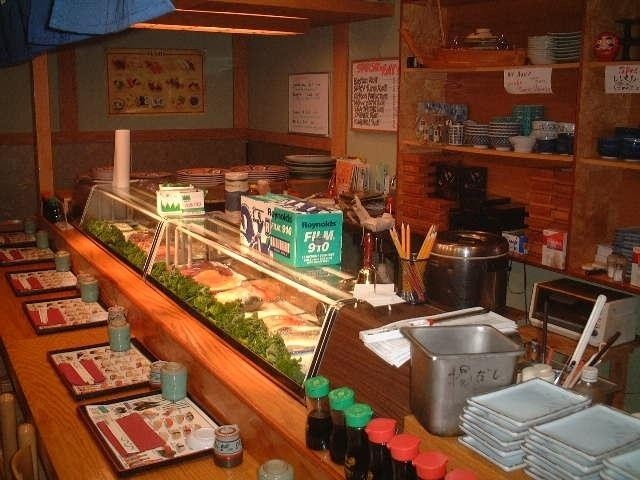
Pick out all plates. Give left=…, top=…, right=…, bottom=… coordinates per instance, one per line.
left=455, top=376, right=639, bottom=479
left=526, top=30, right=582, bottom=65
left=92, top=154, right=337, bottom=191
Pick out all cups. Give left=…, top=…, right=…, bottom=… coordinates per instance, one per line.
left=448, top=124, right=465, bottom=145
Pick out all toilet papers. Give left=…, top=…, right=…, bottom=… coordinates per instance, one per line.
left=112, top=130, right=130, bottom=188
left=225, top=172, right=249, bottom=224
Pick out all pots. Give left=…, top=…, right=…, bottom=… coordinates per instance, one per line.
left=426, top=228, right=511, bottom=318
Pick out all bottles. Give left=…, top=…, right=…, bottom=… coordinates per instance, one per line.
left=574, top=365, right=603, bottom=407
left=343, top=403, right=374, bottom=480
left=107, top=318, right=131, bottom=352
left=108, top=304, right=127, bottom=322
left=23, top=217, right=100, bottom=302
left=213, top=423, right=244, bottom=469
left=160, top=361, right=188, bottom=401
left=364, top=416, right=449, bottom=480
left=606, top=253, right=629, bottom=286
left=151, top=359, right=168, bottom=389
left=303, top=375, right=333, bottom=450
left=328, top=386, right=357, bottom=465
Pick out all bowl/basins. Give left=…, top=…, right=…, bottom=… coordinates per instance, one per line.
left=598, top=125, right=640, bottom=162
left=465, top=104, right=576, bottom=156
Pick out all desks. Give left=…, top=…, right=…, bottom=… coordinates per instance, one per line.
left=515, top=323, right=639, bottom=413
left=0, top=217, right=264, bottom=478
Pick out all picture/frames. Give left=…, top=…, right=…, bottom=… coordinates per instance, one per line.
left=104, top=48, right=208, bottom=118
left=286, top=71, right=330, bottom=137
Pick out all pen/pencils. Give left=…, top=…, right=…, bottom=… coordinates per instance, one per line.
left=389, top=221, right=438, bottom=304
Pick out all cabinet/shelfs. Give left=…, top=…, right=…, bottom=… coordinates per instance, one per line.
left=397, top=0, right=640, bottom=291
